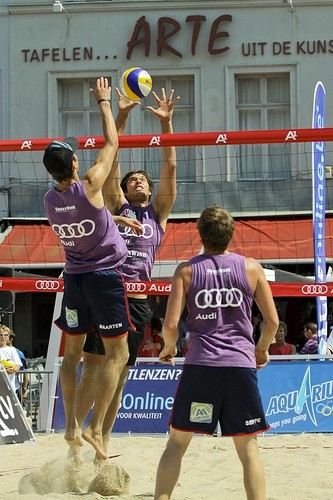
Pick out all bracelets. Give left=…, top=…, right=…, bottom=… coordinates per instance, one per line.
left=98, top=98, right=111, bottom=103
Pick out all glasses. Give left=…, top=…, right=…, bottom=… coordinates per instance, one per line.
left=0, top=333, right=9, bottom=336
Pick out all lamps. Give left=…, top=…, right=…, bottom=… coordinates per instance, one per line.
left=53, top=1, right=69, bottom=16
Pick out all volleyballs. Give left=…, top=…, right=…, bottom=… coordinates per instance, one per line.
left=118, top=67, right=152, bottom=100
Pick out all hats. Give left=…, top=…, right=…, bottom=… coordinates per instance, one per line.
left=44, top=137, right=79, bottom=168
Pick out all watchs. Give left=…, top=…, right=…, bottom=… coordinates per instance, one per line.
left=23, top=387, right=27, bottom=392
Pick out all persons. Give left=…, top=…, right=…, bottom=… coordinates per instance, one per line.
left=154, top=205, right=279, bottom=500
left=142, top=318, right=186, bottom=357
left=0, top=326, right=28, bottom=403
left=68, top=85, right=181, bottom=465
left=297, top=323, right=318, bottom=361
left=42, top=77, right=129, bottom=459
left=269, top=321, right=295, bottom=361
